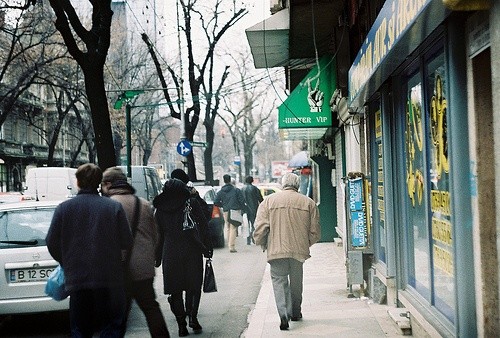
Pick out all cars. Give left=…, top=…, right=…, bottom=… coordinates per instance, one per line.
left=197, top=185, right=226, bottom=249
left=0, top=199, right=71, bottom=314
left=260, top=185, right=279, bottom=201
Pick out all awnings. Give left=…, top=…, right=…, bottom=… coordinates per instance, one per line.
left=287, top=150, right=311, bottom=167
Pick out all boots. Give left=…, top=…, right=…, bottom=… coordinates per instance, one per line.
left=186, top=292, right=202, bottom=330
left=168, top=298, right=189, bottom=337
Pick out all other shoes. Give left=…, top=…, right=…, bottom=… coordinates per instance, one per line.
left=280, top=317, right=289, bottom=331
left=291, top=313, right=302, bottom=322
left=229, top=249, right=237, bottom=253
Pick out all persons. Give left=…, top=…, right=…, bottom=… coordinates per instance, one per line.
left=152, top=168, right=213, bottom=337
left=45, top=164, right=133, bottom=338
left=102, top=167, right=170, bottom=338
left=241, top=175, right=264, bottom=245
left=213, top=174, right=245, bottom=253
left=253, top=173, right=320, bottom=330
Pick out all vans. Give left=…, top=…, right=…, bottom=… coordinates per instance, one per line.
left=24, top=166, right=80, bottom=201
left=114, top=163, right=165, bottom=204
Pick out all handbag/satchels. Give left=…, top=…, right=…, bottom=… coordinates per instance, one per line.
left=227, top=209, right=243, bottom=227
left=202, top=240, right=217, bottom=294
left=182, top=199, right=202, bottom=246
left=45, top=264, right=70, bottom=301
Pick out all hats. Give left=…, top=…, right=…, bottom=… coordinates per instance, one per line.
left=103, top=167, right=127, bottom=182
left=171, top=168, right=189, bottom=184
